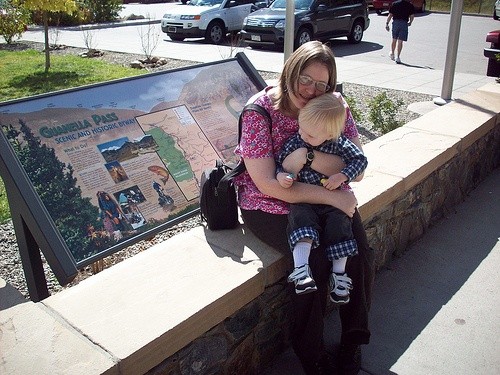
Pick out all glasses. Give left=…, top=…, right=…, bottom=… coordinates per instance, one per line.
left=298, top=76, right=330, bottom=92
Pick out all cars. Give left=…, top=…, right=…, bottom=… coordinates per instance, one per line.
left=373, top=0, right=427, bottom=15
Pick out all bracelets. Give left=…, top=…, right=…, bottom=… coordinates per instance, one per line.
left=386, top=22, right=388, bottom=24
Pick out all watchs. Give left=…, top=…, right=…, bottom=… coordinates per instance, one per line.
left=303, top=146, right=314, bottom=167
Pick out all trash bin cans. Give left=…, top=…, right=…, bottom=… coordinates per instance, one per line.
left=484, top=30, right=500, bottom=77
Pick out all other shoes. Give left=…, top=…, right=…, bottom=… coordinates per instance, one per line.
left=395, top=57, right=400, bottom=63
left=390, top=53, right=395, bottom=60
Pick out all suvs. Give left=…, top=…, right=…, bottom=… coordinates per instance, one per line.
left=161, top=0, right=274, bottom=43
left=240, top=0, right=370, bottom=46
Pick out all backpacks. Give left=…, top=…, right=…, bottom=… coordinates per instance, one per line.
left=198, top=160, right=241, bottom=231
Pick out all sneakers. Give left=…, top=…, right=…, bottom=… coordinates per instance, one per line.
left=286, top=264, right=317, bottom=295
left=330, top=271, right=353, bottom=305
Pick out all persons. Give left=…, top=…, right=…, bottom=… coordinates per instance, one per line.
left=384, top=0, right=415, bottom=64
left=273, top=91, right=370, bottom=305
left=96, top=189, right=128, bottom=235
left=234, top=37, right=371, bottom=375
